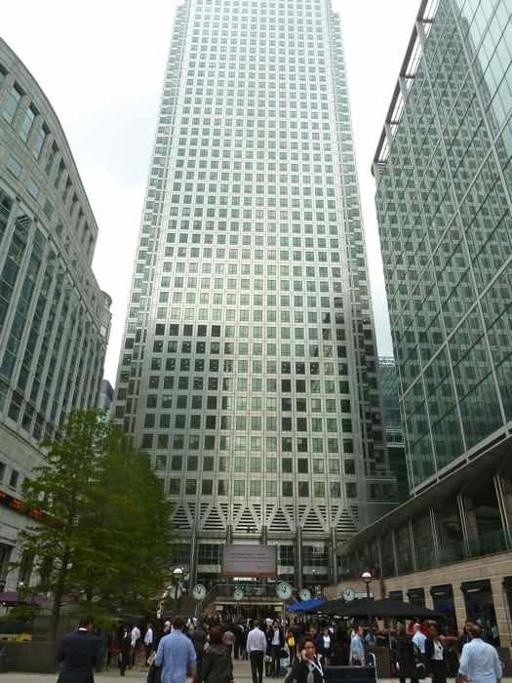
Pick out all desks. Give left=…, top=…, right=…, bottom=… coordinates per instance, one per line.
left=365, top=645, right=392, bottom=678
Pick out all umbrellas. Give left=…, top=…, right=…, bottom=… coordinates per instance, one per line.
left=285, top=597, right=441, bottom=637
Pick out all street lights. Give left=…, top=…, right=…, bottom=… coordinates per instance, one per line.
left=311, top=568, right=316, bottom=585
left=171, top=567, right=184, bottom=612
left=13, top=580, right=24, bottom=601
left=362, top=570, right=374, bottom=643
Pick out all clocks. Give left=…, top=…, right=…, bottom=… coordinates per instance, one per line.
left=192, top=583, right=207, bottom=601
left=169, top=585, right=182, bottom=600
left=299, top=588, right=311, bottom=602
left=275, top=582, right=293, bottom=602
left=341, top=587, right=356, bottom=607
left=232, top=589, right=244, bottom=601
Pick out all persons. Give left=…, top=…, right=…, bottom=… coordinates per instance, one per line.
left=154, top=615, right=198, bottom=682
left=246, top=619, right=268, bottom=682
left=283, top=639, right=327, bottom=682
left=393, top=621, right=502, bottom=682
left=254, top=616, right=366, bottom=679
left=182, top=614, right=254, bottom=682
left=56, top=615, right=103, bottom=682
left=118, top=619, right=173, bottom=676
